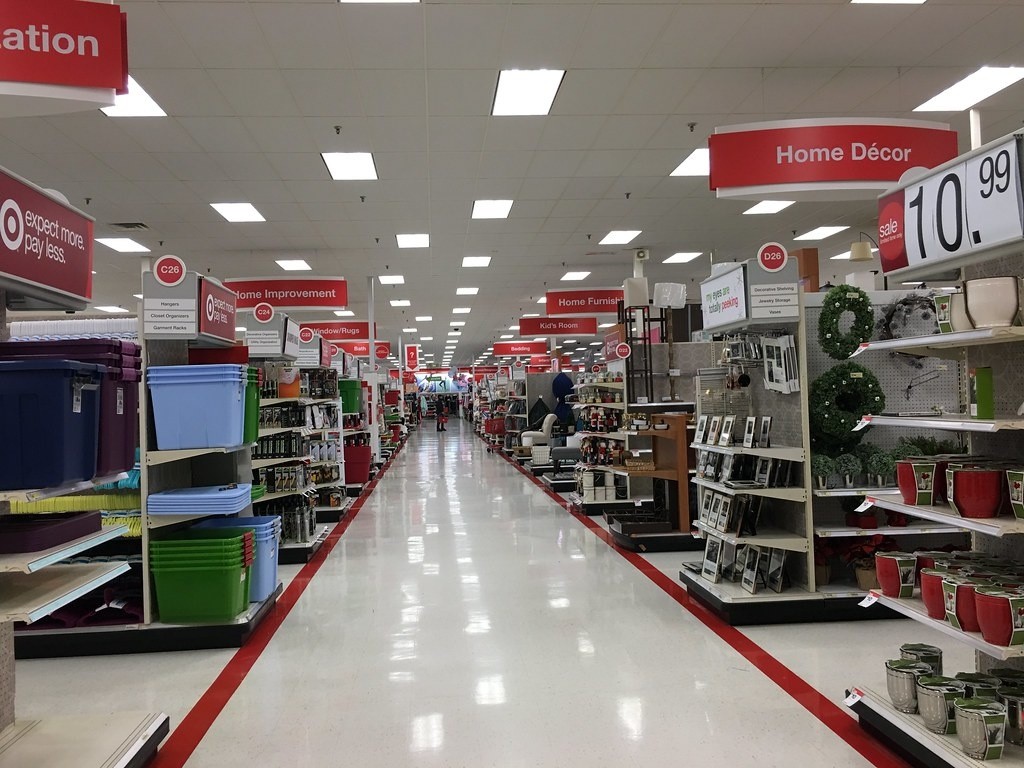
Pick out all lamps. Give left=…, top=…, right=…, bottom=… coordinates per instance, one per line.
left=623, top=278, right=653, bottom=403
left=654, top=282, right=686, bottom=403
left=849, top=232, right=879, bottom=261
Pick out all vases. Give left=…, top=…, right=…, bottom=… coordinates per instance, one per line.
left=854, top=567, right=883, bottom=592
left=816, top=565, right=833, bottom=584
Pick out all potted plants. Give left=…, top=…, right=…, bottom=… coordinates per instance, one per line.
left=812, top=445, right=921, bottom=489
left=837, top=496, right=909, bottom=529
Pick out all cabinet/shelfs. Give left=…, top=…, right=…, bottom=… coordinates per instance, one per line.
left=473, top=374, right=529, bottom=457
left=571, top=344, right=707, bottom=553
left=384, top=403, right=402, bottom=448
left=843, top=131, right=1023, bottom=768
left=308, top=369, right=355, bottom=522
left=260, top=397, right=330, bottom=565
left=0, top=164, right=169, bottom=768
left=678, top=280, right=971, bottom=627
left=136, top=303, right=283, bottom=654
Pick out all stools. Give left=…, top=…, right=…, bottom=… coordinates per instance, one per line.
left=552, top=447, right=581, bottom=477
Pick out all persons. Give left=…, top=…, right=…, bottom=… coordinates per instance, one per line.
left=439, top=376, right=446, bottom=390
left=581, top=408, right=615, bottom=465
left=418, top=374, right=436, bottom=392
left=436, top=394, right=446, bottom=432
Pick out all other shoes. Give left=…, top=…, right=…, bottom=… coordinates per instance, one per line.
left=441, top=428, right=446, bottom=431
left=437, top=428, right=442, bottom=432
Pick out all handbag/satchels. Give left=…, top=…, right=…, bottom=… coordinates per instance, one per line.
left=438, top=414, right=448, bottom=424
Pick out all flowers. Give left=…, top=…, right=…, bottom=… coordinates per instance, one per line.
left=815, top=540, right=834, bottom=565
left=842, top=535, right=899, bottom=569
left=876, top=288, right=940, bottom=370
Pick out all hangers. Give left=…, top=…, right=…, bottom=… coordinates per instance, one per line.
left=10, top=448, right=142, bottom=630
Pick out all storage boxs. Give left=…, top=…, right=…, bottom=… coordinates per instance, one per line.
left=343, top=447, right=372, bottom=483
left=152, top=514, right=282, bottom=624
left=144, top=363, right=262, bottom=450
left=387, top=425, right=401, bottom=442
left=342, top=413, right=366, bottom=497
left=385, top=389, right=400, bottom=405
left=0, top=339, right=143, bottom=490
left=250, top=370, right=346, bottom=545
left=338, top=380, right=360, bottom=412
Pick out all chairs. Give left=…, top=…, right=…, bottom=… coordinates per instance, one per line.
left=521, top=414, right=558, bottom=446
